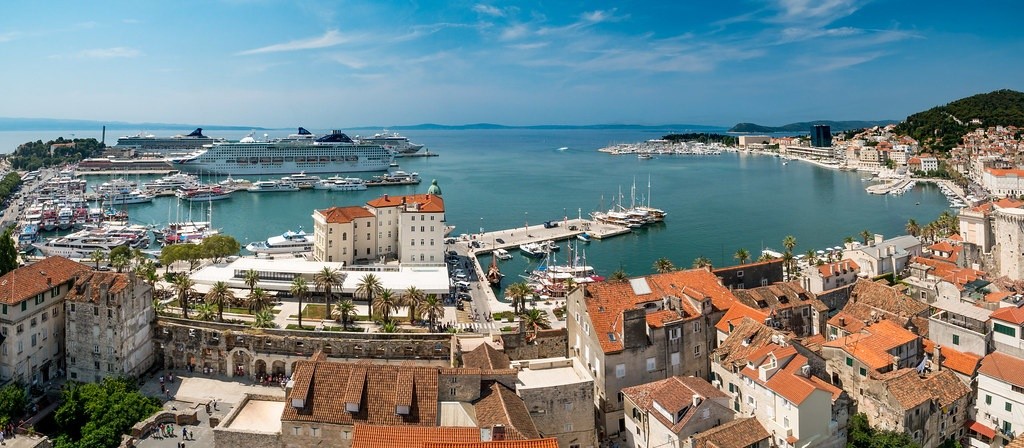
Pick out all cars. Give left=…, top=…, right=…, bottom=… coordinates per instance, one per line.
left=444, top=238, right=480, bottom=304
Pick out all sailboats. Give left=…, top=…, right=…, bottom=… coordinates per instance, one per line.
left=486, top=239, right=606, bottom=301
left=586, top=172, right=668, bottom=228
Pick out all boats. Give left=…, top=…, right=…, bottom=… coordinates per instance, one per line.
left=599, top=140, right=739, bottom=159
left=360, top=132, right=425, bottom=153
left=391, top=161, right=399, bottom=167
left=577, top=234, right=591, bottom=242
left=866, top=180, right=968, bottom=210
left=15, top=163, right=423, bottom=260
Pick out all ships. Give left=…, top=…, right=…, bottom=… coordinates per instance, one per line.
left=166, top=134, right=397, bottom=173
